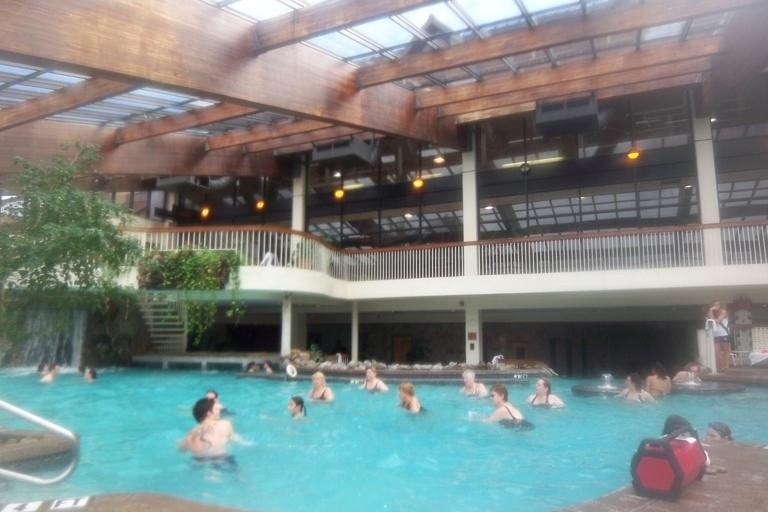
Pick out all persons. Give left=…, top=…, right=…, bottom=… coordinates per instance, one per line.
left=525, top=375, right=564, bottom=409
left=701, top=420, right=733, bottom=444
left=247, top=361, right=257, bottom=373
left=468, top=382, right=533, bottom=434
left=263, top=359, right=275, bottom=373
left=286, top=395, right=308, bottom=420
left=38, top=362, right=48, bottom=376
left=84, top=366, right=97, bottom=381
left=612, top=370, right=654, bottom=404
left=355, top=365, right=389, bottom=395
left=38, top=361, right=62, bottom=385
left=76, top=363, right=86, bottom=377
left=706, top=299, right=732, bottom=371
left=643, top=359, right=675, bottom=402
left=306, top=371, right=336, bottom=405
left=392, top=381, right=430, bottom=414
left=174, top=395, right=251, bottom=474
left=205, top=390, right=236, bottom=419
left=459, top=369, right=487, bottom=398
